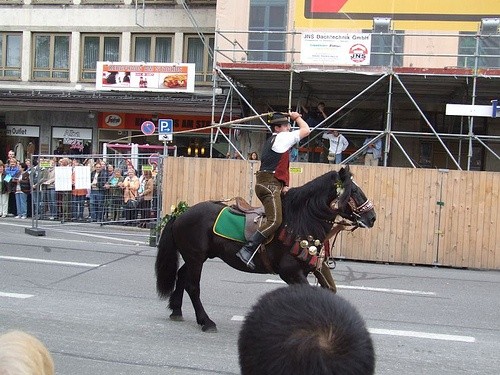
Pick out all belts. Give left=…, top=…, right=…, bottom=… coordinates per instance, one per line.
left=365, top=151, right=374, bottom=155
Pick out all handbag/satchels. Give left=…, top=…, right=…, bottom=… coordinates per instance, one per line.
left=327, top=153, right=336, bottom=161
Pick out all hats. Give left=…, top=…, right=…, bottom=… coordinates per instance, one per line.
left=267, top=112, right=292, bottom=124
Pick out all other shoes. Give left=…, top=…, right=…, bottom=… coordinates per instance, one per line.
left=35, top=216, right=85, bottom=223
left=2, top=212, right=7, bottom=217
left=15, top=215, right=21, bottom=220
left=21, top=215, right=27, bottom=220
left=138, top=222, right=148, bottom=228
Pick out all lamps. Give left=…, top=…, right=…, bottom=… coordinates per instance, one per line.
left=372, top=17, right=391, bottom=34
left=480, top=18, right=500, bottom=36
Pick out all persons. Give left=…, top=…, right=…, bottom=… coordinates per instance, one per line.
left=5, top=158, right=20, bottom=217
left=235, top=112, right=310, bottom=270
left=0, top=164, right=11, bottom=218
left=12, top=163, right=29, bottom=219
left=363, top=136, right=382, bottom=166
left=0, top=150, right=20, bottom=167
left=301, top=101, right=328, bottom=127
left=233, top=150, right=258, bottom=160
left=322, top=130, right=349, bottom=164
left=25, top=157, right=158, bottom=229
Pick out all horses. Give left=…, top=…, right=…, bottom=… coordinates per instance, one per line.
left=153, top=165, right=377, bottom=332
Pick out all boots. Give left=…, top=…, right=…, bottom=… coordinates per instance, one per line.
left=236, top=229, right=267, bottom=270
